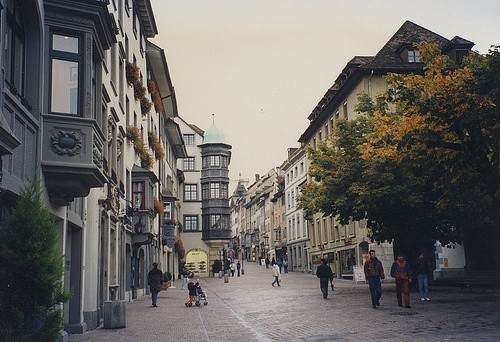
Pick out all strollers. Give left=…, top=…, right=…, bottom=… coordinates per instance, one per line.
left=183, top=283, right=208, bottom=307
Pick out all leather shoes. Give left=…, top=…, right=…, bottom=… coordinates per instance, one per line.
left=372, top=302, right=380, bottom=308
left=323, top=292, right=327, bottom=299
left=398, top=301, right=411, bottom=308
left=152, top=303, right=158, bottom=307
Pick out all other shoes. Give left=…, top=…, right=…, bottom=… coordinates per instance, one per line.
left=272, top=283, right=280, bottom=287
left=259, top=263, right=288, bottom=274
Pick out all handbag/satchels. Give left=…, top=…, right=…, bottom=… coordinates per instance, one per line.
left=331, top=281, right=334, bottom=291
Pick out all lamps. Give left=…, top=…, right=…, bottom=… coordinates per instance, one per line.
left=134, top=215, right=146, bottom=235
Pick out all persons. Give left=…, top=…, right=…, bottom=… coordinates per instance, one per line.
left=364, top=249, right=385, bottom=308
left=316, top=258, right=334, bottom=299
left=184, top=281, right=198, bottom=306
left=411, top=252, right=432, bottom=301
left=147, top=262, right=164, bottom=307
left=210, top=252, right=290, bottom=287
left=390, top=252, right=411, bottom=308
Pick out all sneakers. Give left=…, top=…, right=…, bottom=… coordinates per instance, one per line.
left=420, top=297, right=431, bottom=301
